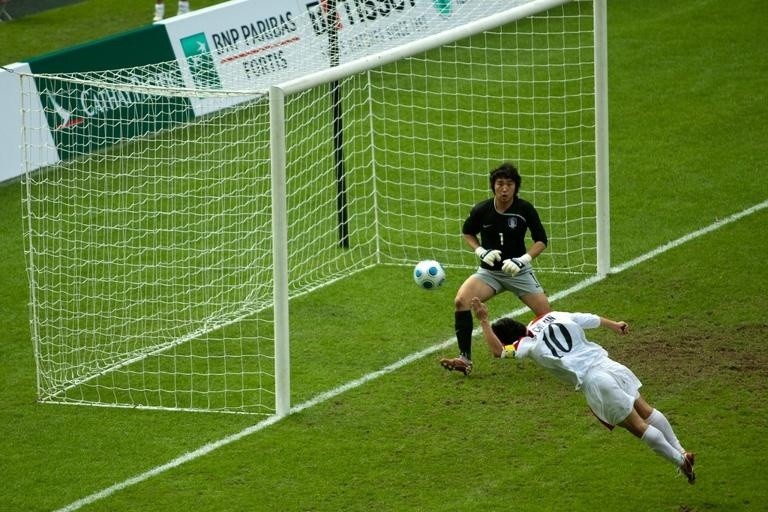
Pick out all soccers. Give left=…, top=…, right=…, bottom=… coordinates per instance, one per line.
left=414, top=260, right=445, bottom=289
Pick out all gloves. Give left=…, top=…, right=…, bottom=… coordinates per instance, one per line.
left=475, top=247, right=532, bottom=278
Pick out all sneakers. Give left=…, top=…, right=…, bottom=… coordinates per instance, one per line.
left=678, top=452, right=695, bottom=484
left=440, top=355, right=473, bottom=375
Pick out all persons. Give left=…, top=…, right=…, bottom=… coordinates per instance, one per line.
left=151, top=0, right=191, bottom=23
left=469, top=295, right=696, bottom=485
left=438, top=164, right=551, bottom=374
